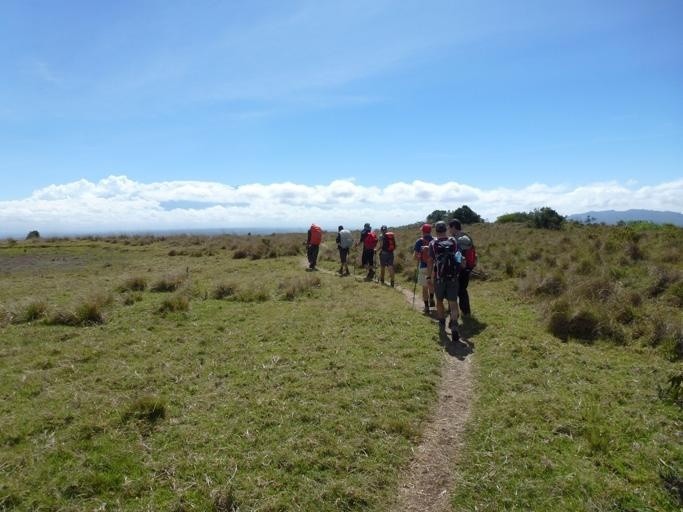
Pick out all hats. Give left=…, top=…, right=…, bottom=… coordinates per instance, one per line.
left=381, top=225, right=386, bottom=230
left=420, top=223, right=432, bottom=232
left=435, top=220, right=446, bottom=232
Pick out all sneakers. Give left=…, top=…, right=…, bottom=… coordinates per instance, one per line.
left=339, top=269, right=350, bottom=275
left=424, top=300, right=435, bottom=312
left=440, top=324, right=447, bottom=336
left=381, top=278, right=394, bottom=286
left=452, top=326, right=459, bottom=341
left=366, top=270, right=376, bottom=278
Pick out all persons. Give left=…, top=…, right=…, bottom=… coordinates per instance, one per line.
left=306, top=224, right=320, bottom=269
left=425, top=220, right=460, bottom=342
left=374, top=225, right=395, bottom=288
left=448, top=219, right=472, bottom=319
left=412, top=224, right=435, bottom=313
left=336, top=226, right=350, bottom=275
left=355, top=223, right=376, bottom=279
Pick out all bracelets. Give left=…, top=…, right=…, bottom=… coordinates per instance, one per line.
left=426, top=277, right=431, bottom=280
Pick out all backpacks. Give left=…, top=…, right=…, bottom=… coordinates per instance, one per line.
left=310, top=224, right=321, bottom=245
left=385, top=231, right=395, bottom=250
left=365, top=232, right=378, bottom=249
left=420, top=237, right=430, bottom=263
left=435, top=239, right=458, bottom=280
